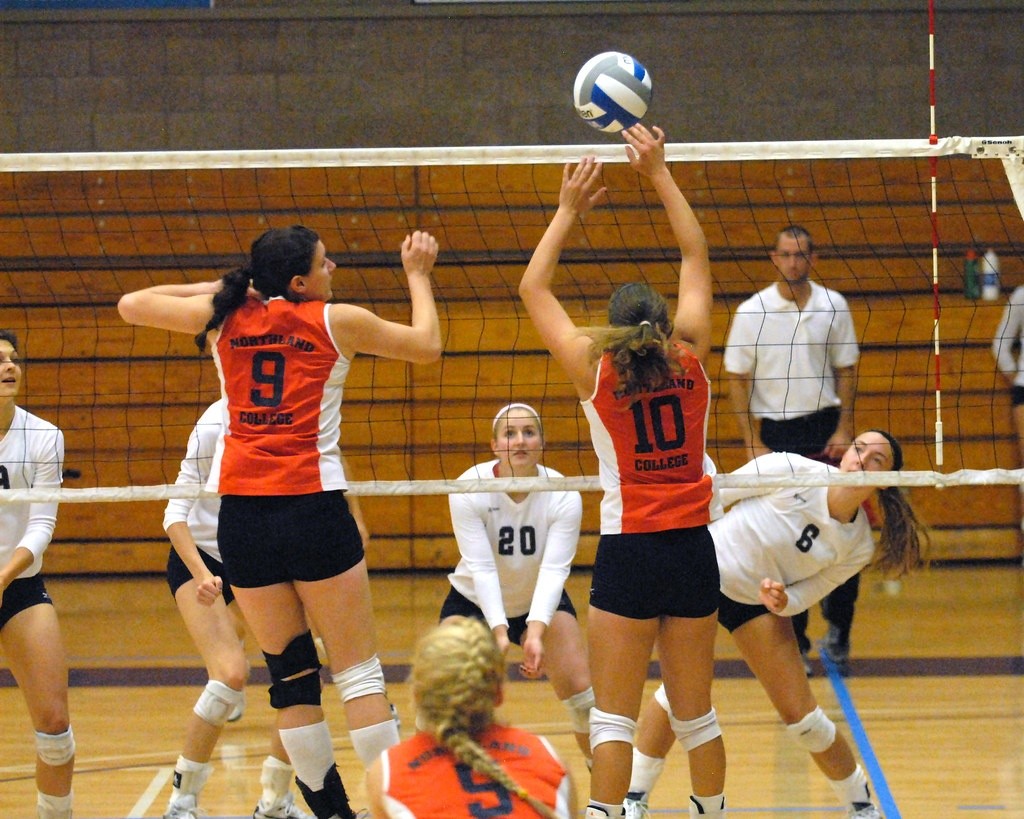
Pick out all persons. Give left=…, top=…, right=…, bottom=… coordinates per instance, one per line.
left=160, top=397, right=311, bottom=819
left=725, top=227, right=860, bottom=679
left=990, top=283, right=1024, bottom=470
left=0, top=331, right=74, bottom=819
left=364, top=616, right=577, bottom=819
left=437, top=403, right=603, bottom=773
left=119, top=225, right=447, bottom=818
left=619, top=429, right=927, bottom=819
left=520, top=121, right=728, bottom=819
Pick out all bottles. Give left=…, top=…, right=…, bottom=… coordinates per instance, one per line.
left=965, top=250, right=981, bottom=299
left=982, top=249, right=999, bottom=301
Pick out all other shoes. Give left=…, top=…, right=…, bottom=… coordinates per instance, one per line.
left=825, top=620, right=848, bottom=668
left=801, top=647, right=813, bottom=679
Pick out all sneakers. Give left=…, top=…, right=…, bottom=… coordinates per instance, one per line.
left=164, top=805, right=197, bottom=819
left=253, top=788, right=309, bottom=819
left=621, top=792, right=649, bottom=819
left=847, top=799, right=881, bottom=819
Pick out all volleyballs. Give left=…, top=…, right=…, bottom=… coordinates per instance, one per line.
left=572, top=51, right=653, bottom=132
